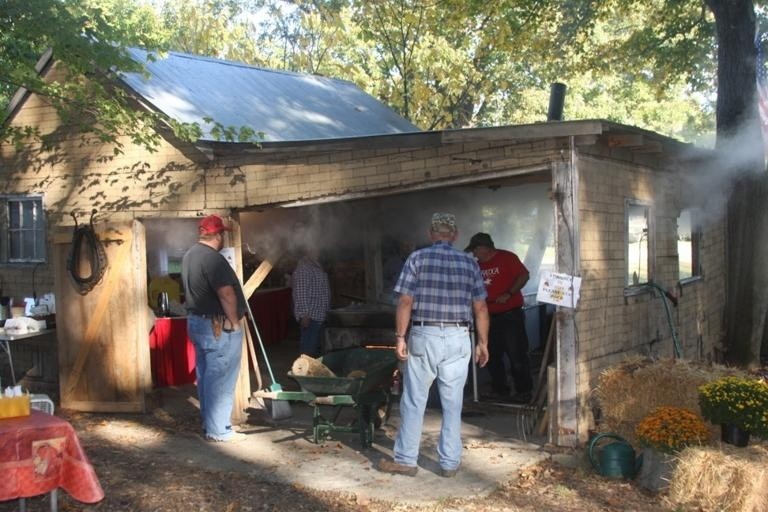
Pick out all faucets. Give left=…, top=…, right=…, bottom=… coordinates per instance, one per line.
left=157, top=289, right=170, bottom=317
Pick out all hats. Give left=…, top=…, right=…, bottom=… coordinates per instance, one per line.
left=463, top=233, right=493, bottom=252
left=431, top=213, right=456, bottom=232
left=197, top=215, right=234, bottom=235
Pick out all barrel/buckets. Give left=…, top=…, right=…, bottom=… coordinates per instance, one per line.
left=635, top=445, right=675, bottom=493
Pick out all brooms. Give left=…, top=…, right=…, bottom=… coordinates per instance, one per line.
left=244, top=293, right=293, bottom=420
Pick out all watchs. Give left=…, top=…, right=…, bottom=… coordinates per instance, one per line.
left=393, top=332, right=406, bottom=338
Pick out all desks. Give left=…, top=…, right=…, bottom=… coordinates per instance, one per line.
left=0, top=327, right=57, bottom=388
left=0, top=408, right=106, bottom=511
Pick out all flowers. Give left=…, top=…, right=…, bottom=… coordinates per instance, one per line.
left=637, top=407, right=710, bottom=453
left=699, top=375, right=768, bottom=434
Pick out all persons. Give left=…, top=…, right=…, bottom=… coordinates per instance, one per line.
left=377, top=212, right=489, bottom=477
left=181, top=215, right=247, bottom=442
left=293, top=241, right=329, bottom=406
left=463, top=233, right=533, bottom=404
left=383, top=234, right=410, bottom=328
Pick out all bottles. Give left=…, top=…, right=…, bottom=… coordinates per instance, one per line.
left=0, top=297, right=10, bottom=329
left=157, top=287, right=170, bottom=314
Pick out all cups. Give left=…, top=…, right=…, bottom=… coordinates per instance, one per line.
left=24, top=293, right=55, bottom=318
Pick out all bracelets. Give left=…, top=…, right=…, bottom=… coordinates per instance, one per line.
left=507, top=291, right=513, bottom=297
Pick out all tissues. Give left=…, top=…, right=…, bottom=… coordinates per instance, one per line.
left=0, top=384, right=32, bottom=420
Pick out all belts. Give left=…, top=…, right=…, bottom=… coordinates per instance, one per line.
left=413, top=321, right=468, bottom=327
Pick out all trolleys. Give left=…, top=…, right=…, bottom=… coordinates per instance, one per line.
left=253, top=349, right=402, bottom=449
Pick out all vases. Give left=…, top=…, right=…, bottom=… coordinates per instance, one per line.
left=640, top=447, right=675, bottom=494
left=722, top=423, right=748, bottom=447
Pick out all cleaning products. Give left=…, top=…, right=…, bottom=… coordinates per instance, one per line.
left=24, top=297, right=37, bottom=317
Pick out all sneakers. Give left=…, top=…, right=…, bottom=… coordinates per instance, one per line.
left=377, top=457, right=417, bottom=477
left=208, top=432, right=248, bottom=444
